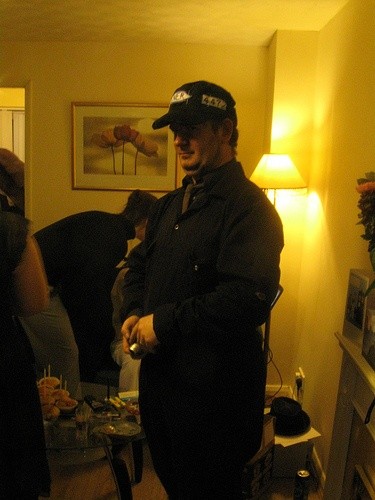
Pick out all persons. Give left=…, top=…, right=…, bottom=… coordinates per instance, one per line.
left=20, top=190, right=157, bottom=400
left=120, top=80, right=284, bottom=500
left=0, top=210, right=51, bottom=500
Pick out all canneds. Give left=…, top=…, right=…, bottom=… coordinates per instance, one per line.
left=294, top=470, right=310, bottom=500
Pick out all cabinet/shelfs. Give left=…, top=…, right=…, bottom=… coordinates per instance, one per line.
left=321, top=331, right=375, bottom=500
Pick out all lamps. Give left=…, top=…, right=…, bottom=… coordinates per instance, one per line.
left=248, top=153, right=307, bottom=362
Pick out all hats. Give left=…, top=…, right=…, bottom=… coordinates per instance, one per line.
left=153, top=81, right=236, bottom=129
left=267, top=395, right=310, bottom=436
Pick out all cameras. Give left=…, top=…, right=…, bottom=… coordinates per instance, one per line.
left=126, top=335, right=148, bottom=357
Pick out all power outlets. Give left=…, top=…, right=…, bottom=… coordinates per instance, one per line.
left=298, top=367, right=305, bottom=392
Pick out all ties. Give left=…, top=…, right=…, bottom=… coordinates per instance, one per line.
left=180, top=181, right=195, bottom=216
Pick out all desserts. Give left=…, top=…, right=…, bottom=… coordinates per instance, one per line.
left=37, top=377, right=78, bottom=416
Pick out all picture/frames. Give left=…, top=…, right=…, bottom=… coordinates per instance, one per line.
left=71, top=101, right=178, bottom=193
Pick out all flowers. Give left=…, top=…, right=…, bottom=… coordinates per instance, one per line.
left=355, top=172, right=375, bottom=273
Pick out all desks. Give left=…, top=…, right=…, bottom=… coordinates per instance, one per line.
left=40, top=402, right=148, bottom=500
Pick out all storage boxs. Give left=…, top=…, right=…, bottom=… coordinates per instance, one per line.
left=241, top=414, right=275, bottom=500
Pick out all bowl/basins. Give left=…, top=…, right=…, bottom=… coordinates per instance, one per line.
left=38, top=376, right=78, bottom=423
